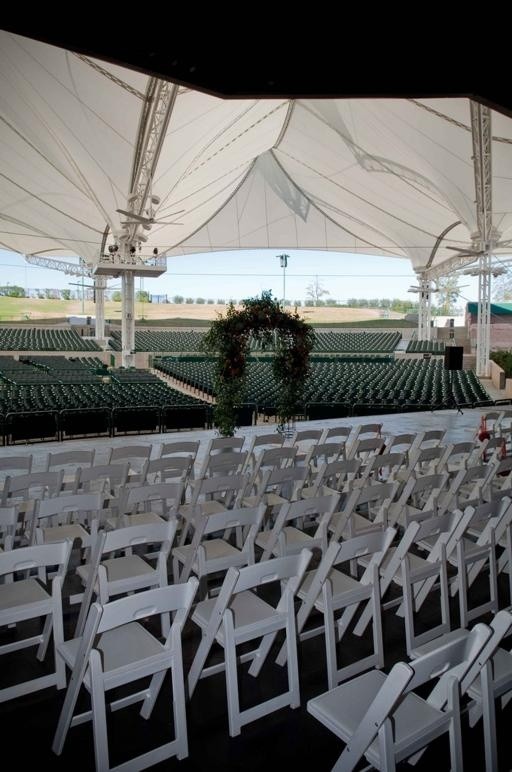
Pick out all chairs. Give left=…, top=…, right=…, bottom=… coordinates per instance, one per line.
left=108, top=329, right=403, bottom=352
left=0, top=355, right=220, bottom=418
left=0, top=329, right=103, bottom=352
left=219, top=354, right=511, bottom=424
left=405, top=339, right=446, bottom=354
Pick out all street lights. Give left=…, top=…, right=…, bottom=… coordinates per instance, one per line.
left=277, top=253, right=291, bottom=314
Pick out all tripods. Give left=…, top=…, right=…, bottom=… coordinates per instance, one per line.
left=431, top=376, right=464, bottom=415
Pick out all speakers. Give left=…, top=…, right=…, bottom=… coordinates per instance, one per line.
left=443, top=345, right=465, bottom=371
